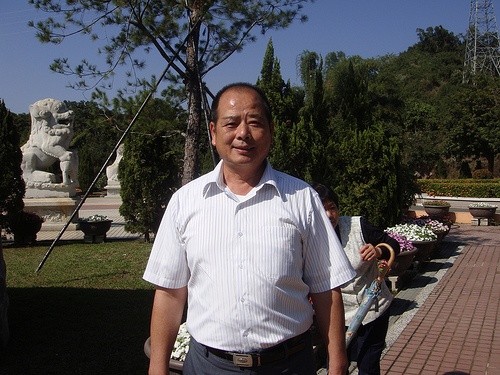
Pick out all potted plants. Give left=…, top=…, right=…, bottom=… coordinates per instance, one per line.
left=10, top=212, right=45, bottom=242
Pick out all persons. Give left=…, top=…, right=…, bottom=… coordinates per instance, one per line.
left=310, top=184, right=400, bottom=375
left=142, top=84, right=360, bottom=375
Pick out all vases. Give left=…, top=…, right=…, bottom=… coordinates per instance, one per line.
left=390, top=246, right=418, bottom=275
left=424, top=206, right=450, bottom=216
left=79, top=220, right=113, bottom=236
left=144, top=336, right=185, bottom=375
left=412, top=239, right=438, bottom=255
left=433, top=228, right=451, bottom=243
left=469, top=207, right=497, bottom=217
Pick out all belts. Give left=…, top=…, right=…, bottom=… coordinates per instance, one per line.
left=201, top=333, right=312, bottom=369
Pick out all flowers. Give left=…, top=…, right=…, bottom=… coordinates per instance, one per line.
left=423, top=200, right=450, bottom=206
left=469, top=202, right=498, bottom=207
left=81, top=214, right=110, bottom=222
left=170, top=321, right=191, bottom=363
left=383, top=215, right=453, bottom=252
